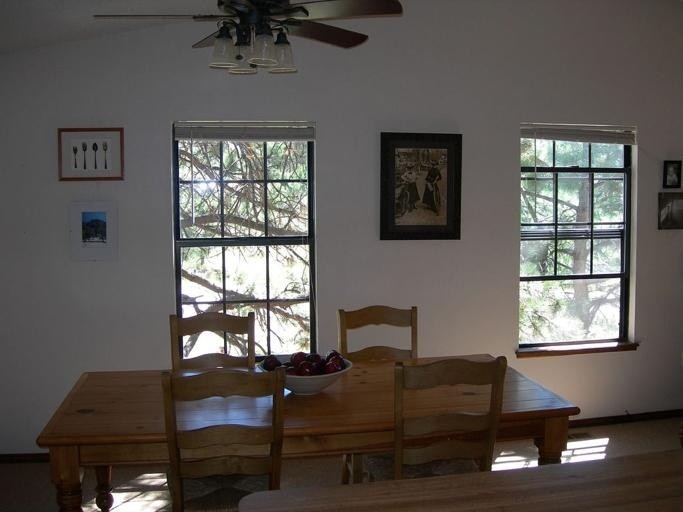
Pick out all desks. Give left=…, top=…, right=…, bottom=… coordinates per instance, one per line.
left=37, top=354, right=581, bottom=512
left=238, top=445, right=683, bottom=512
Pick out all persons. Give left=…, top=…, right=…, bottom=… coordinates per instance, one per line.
left=666, top=166, right=678, bottom=185
left=421, top=158, right=442, bottom=216
left=400, top=163, right=421, bottom=213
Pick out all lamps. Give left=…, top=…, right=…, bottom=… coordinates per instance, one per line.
left=208, top=21, right=296, bottom=74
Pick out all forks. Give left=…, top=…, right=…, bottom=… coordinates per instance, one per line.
left=73, top=140, right=110, bottom=171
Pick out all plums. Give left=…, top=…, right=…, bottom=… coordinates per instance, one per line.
left=264, top=351, right=345, bottom=375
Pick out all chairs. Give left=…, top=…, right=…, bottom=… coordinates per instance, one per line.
left=338, top=305, right=417, bottom=484
left=352, top=356, right=506, bottom=485
left=169, top=312, right=255, bottom=369
left=161, top=367, right=286, bottom=512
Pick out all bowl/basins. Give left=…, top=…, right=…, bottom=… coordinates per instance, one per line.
left=256, top=350, right=354, bottom=397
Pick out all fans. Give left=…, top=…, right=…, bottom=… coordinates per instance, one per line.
left=92, top=0, right=404, bottom=49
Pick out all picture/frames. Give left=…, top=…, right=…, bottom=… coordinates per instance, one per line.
left=58, top=128, right=124, bottom=181
left=380, top=132, right=462, bottom=240
left=662, top=160, right=682, bottom=188
